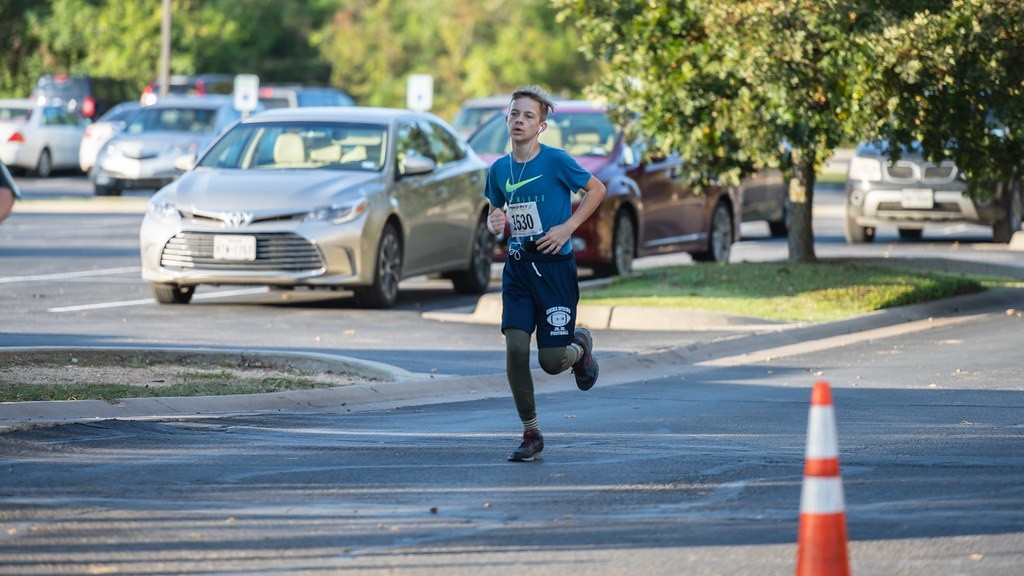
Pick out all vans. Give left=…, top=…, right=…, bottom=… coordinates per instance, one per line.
left=29, top=71, right=128, bottom=123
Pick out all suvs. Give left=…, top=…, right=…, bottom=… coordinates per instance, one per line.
left=845, top=82, right=1024, bottom=245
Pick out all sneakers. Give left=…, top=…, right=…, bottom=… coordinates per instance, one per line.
left=571, top=327, right=599, bottom=391
left=507, top=430, right=545, bottom=461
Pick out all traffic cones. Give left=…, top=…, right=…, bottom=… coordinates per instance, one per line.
left=790, top=381, right=856, bottom=576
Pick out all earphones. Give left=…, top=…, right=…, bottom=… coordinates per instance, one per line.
left=538, top=127, right=542, bottom=134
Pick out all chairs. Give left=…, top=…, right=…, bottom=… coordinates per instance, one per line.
left=273, top=132, right=304, bottom=167
left=574, top=129, right=600, bottom=147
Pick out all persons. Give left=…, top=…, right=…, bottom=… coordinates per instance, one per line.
left=484, top=88, right=607, bottom=463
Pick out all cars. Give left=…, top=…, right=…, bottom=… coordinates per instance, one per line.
left=0, top=93, right=92, bottom=179
left=78, top=69, right=361, bottom=196
left=727, top=132, right=792, bottom=239
left=136, top=103, right=497, bottom=309
left=462, top=98, right=743, bottom=283
left=447, top=94, right=515, bottom=144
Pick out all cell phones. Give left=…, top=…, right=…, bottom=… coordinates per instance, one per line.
left=526, top=241, right=545, bottom=253
